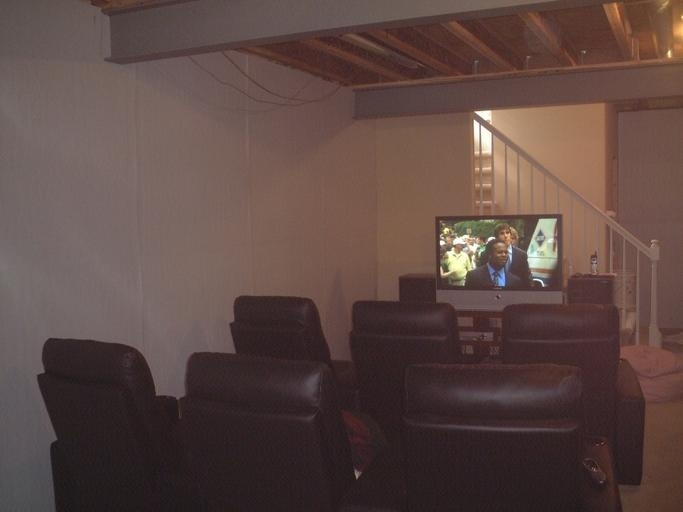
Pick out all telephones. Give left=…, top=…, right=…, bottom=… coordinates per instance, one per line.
left=590, top=250, right=598, bottom=276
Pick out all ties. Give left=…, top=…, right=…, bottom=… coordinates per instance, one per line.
left=493, top=272, right=500, bottom=287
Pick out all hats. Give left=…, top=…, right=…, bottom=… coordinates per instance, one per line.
left=452, top=238, right=465, bottom=245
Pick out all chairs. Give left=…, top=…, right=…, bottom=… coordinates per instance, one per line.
left=350, top=301, right=459, bottom=370
left=180, top=351, right=397, bottom=510
left=398, top=359, right=624, bottom=511
left=502, top=301, right=644, bottom=485
left=35, top=337, right=179, bottom=511
left=231, top=294, right=359, bottom=403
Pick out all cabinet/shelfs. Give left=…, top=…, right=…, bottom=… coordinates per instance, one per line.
left=450, top=309, right=502, bottom=346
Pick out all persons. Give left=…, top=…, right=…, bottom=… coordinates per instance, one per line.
left=440, top=223, right=534, bottom=289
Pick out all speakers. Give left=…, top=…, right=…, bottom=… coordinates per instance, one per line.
left=567, top=274, right=614, bottom=304
left=435, top=214, right=563, bottom=312
left=398, top=273, right=436, bottom=303
